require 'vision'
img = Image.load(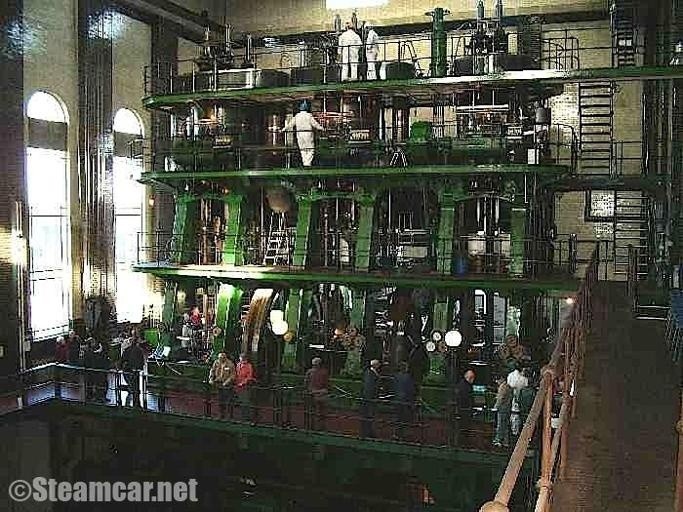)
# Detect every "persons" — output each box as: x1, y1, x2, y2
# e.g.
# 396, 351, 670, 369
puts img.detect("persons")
363, 22, 380, 79
278, 102, 326, 170
337, 24, 364, 83
56, 313, 535, 449
